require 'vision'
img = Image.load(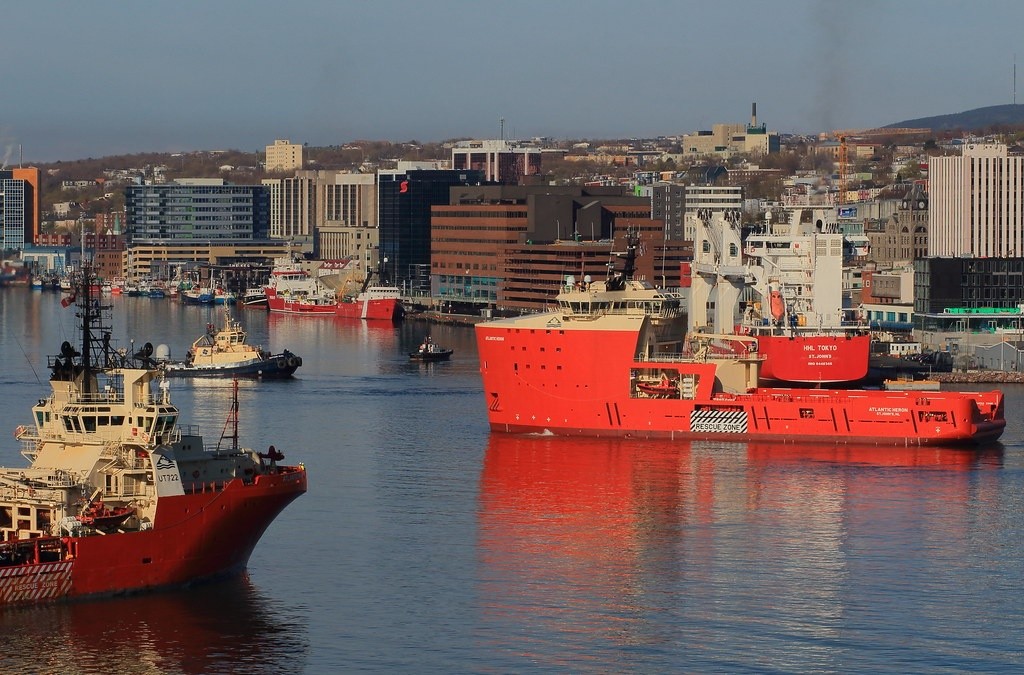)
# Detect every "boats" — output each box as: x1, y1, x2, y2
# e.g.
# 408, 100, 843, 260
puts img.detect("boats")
409, 339, 455, 360
473, 225, 1008, 446
0, 252, 403, 609
